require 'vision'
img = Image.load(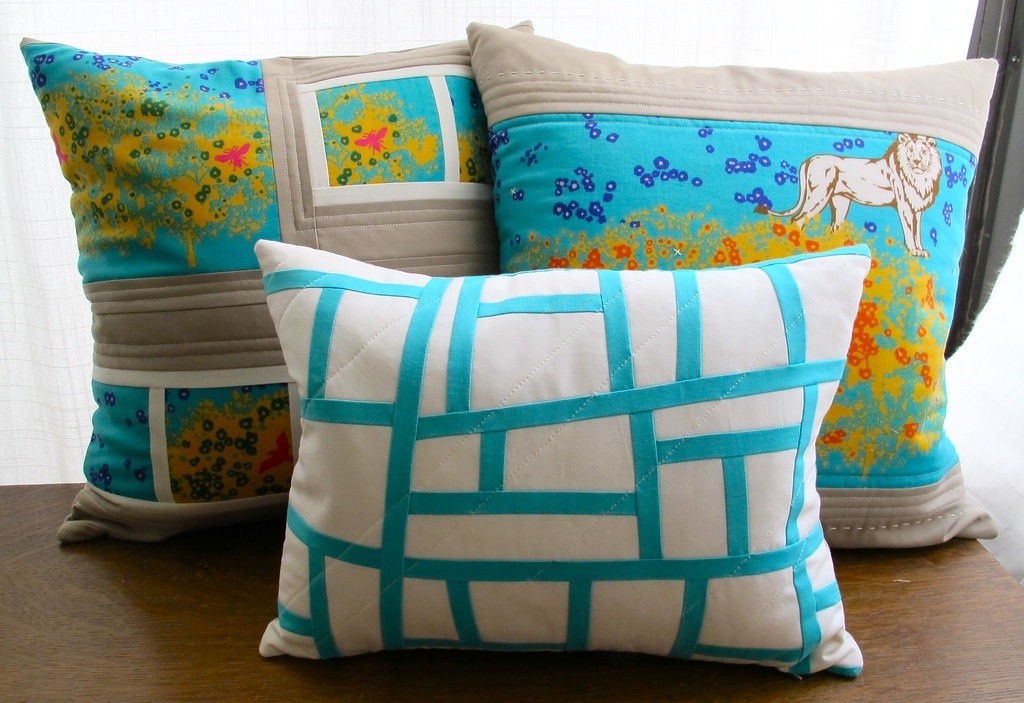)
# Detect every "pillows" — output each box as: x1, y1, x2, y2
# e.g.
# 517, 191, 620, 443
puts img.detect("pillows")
254, 238, 871, 683
16, 16, 536, 548
464, 23, 1002, 552
